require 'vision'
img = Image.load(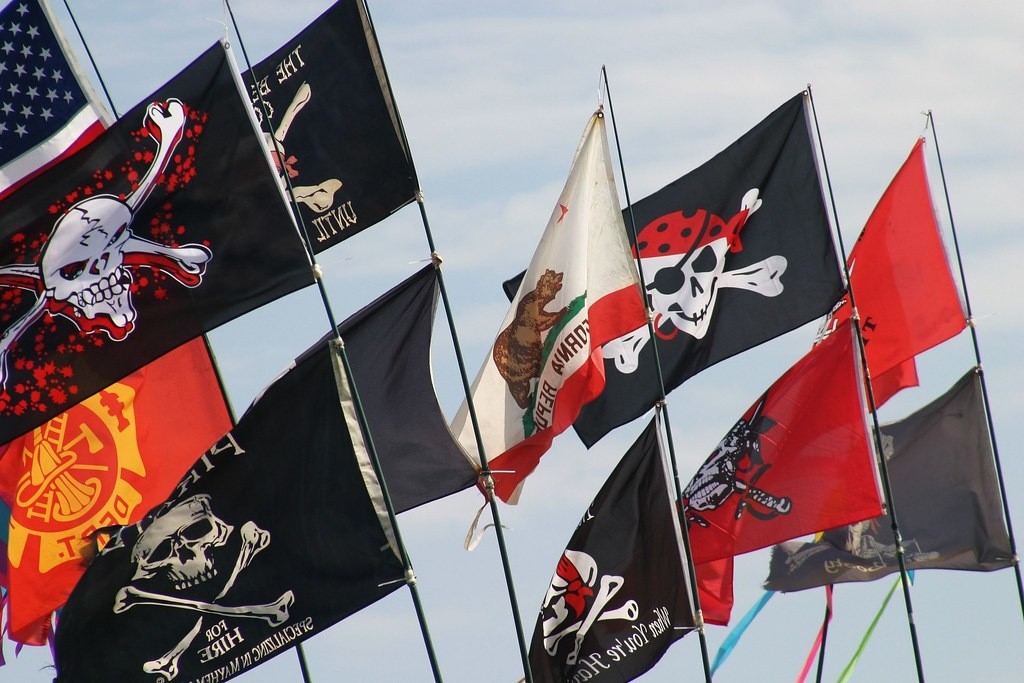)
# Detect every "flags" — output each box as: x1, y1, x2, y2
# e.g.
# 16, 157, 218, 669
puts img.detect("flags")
812, 137, 969, 413
0, 0, 115, 200
0, 334, 235, 647
240, 0, 419, 256
763, 367, 1024, 594
323, 262, 486, 515
502, 90, 846, 450
675, 319, 886, 627
450, 110, 648, 505
528, 415, 697, 683
56, 334, 406, 683
0, 39, 316, 447
